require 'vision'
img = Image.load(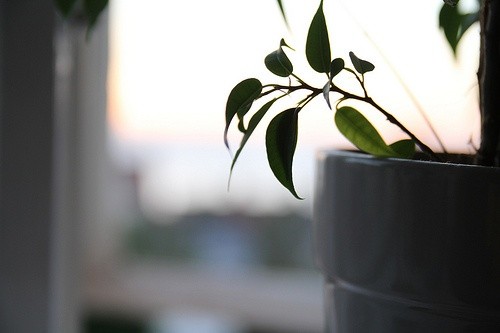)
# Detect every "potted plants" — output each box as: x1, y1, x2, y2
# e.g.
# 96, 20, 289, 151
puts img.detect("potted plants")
224, 0, 498, 333
